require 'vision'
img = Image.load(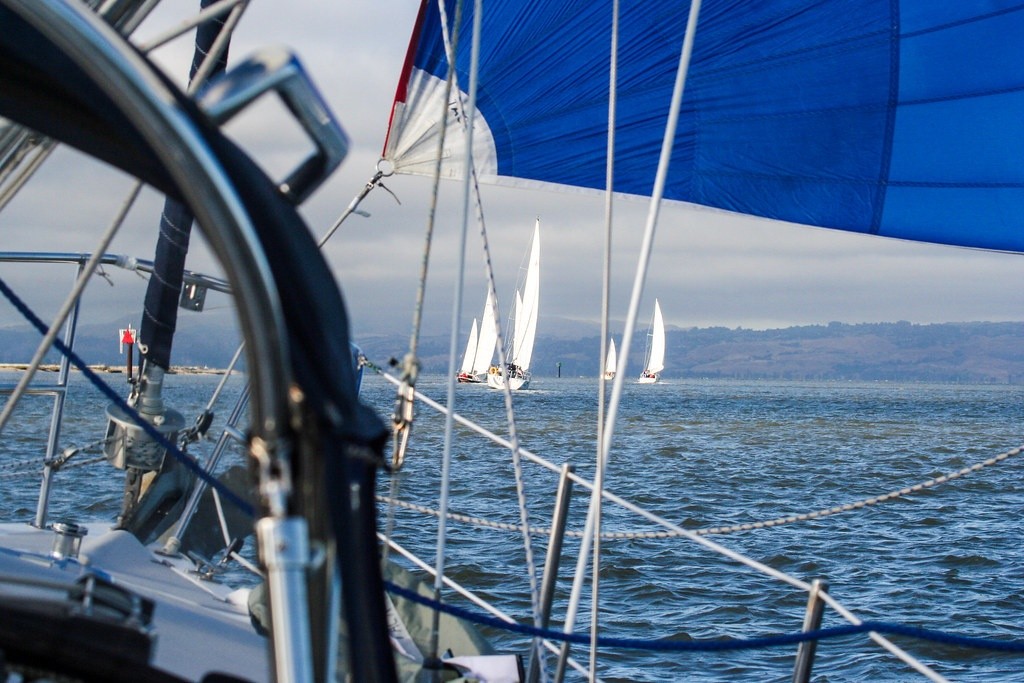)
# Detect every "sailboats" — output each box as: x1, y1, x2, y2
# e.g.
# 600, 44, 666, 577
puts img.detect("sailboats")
485, 216, 541, 390
632, 297, 666, 384
456, 288, 497, 383
604, 335, 617, 379
